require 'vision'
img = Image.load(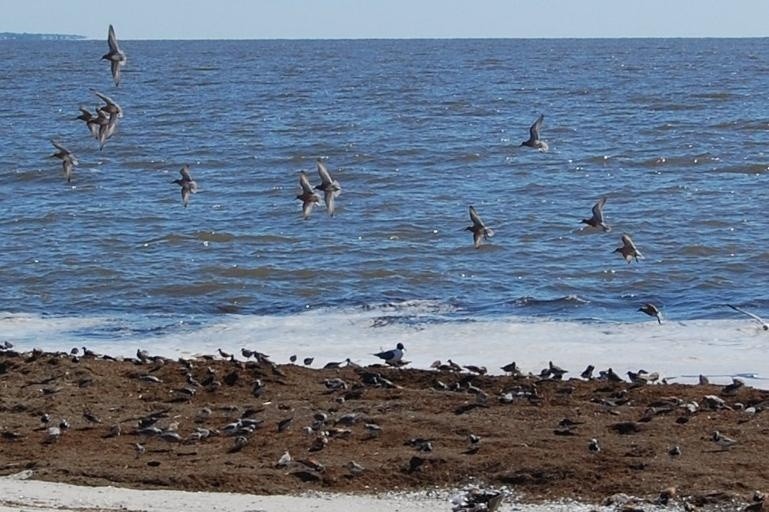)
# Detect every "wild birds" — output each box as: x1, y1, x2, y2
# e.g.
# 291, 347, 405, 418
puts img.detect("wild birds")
170, 167, 199, 208
291, 160, 342, 217
1, 342, 769, 472
40, 25, 127, 185
518, 113, 550, 154
463, 205, 495, 249
726, 303, 768, 331
636, 302, 662, 326
610, 234, 644, 265
578, 197, 612, 234
